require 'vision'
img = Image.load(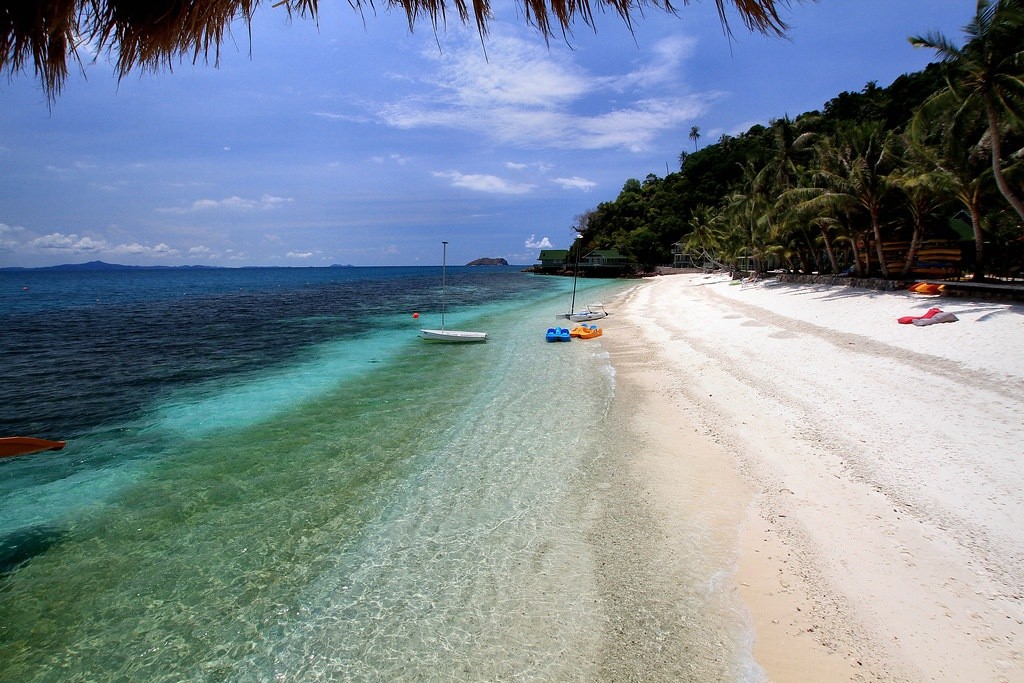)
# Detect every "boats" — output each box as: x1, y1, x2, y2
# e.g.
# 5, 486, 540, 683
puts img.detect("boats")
0, 437, 66, 459
556, 312, 606, 321
419, 328, 486, 342
